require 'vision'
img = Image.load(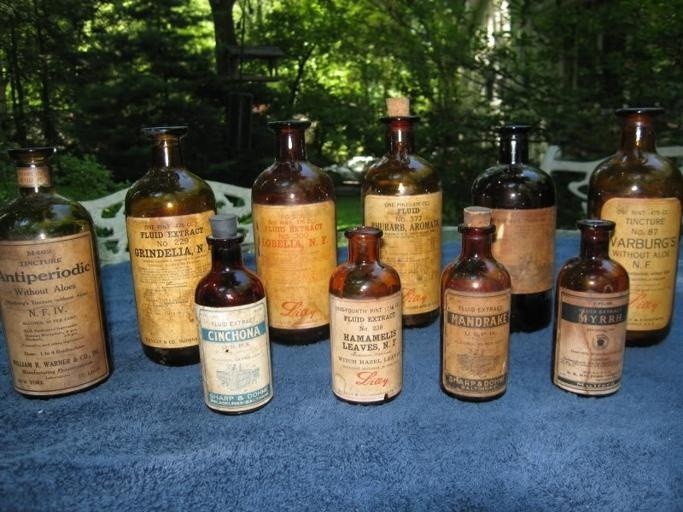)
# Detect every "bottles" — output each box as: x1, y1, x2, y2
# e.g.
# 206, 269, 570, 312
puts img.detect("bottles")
589, 108, 682, 348
251, 120, 340, 345
363, 99, 444, 329
554, 219, 630, 398
194, 217, 274, 414
442, 209, 511, 402
328, 227, 403, 405
123, 127, 217, 365
1, 145, 113, 398
472, 126, 557, 334
